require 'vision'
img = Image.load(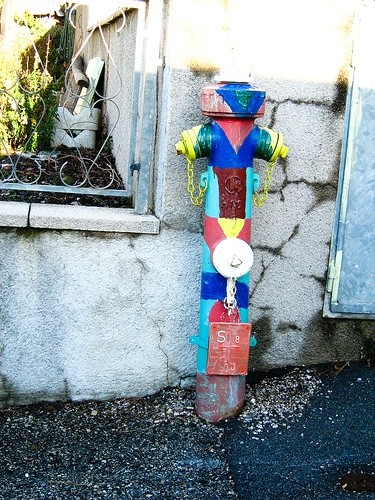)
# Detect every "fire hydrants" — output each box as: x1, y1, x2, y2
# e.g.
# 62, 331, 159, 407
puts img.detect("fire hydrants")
174, 81, 289, 424
